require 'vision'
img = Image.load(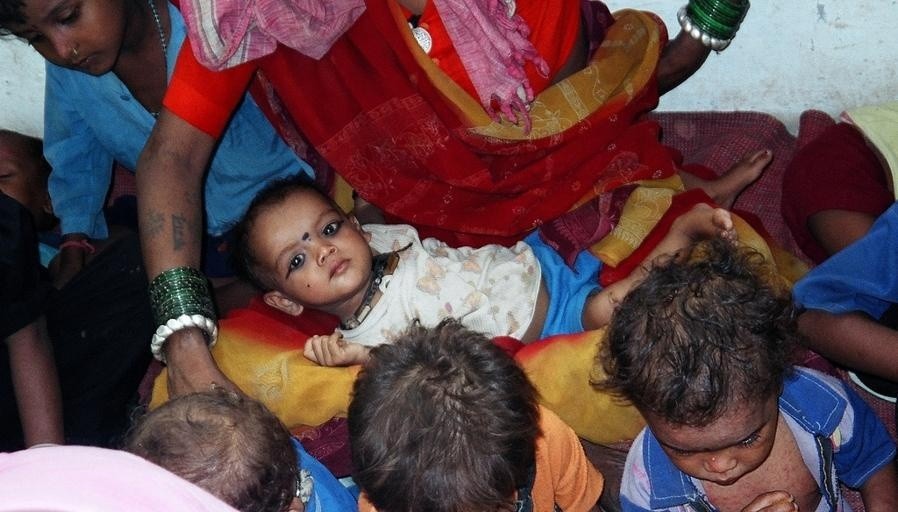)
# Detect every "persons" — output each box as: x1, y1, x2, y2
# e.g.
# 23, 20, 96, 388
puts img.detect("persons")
3, 1, 898, 512
